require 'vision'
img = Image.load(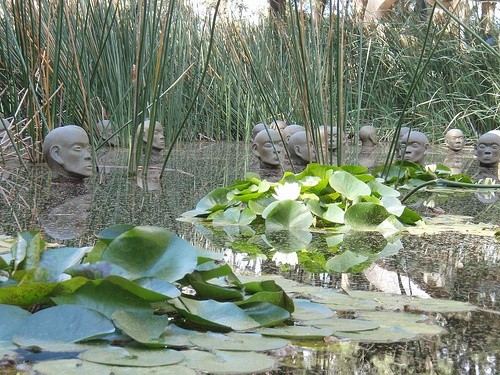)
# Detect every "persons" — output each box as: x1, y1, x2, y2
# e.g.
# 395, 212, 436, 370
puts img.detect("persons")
42, 126, 94, 185
137, 163, 163, 190
96, 147, 120, 174
39, 186, 94, 240
136, 119, 165, 163
475, 166, 500, 203
96, 120, 120, 147
253, 120, 345, 172
359, 125, 380, 148
391, 126, 412, 158
398, 131, 431, 169
357, 148, 378, 167
0, 118, 15, 156
442, 152, 466, 176
444, 129, 466, 151
474, 130, 500, 167
